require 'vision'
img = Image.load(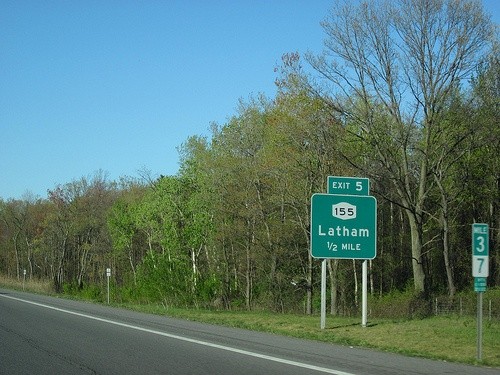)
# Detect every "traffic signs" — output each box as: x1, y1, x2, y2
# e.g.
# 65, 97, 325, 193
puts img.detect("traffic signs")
312, 175, 377, 261
